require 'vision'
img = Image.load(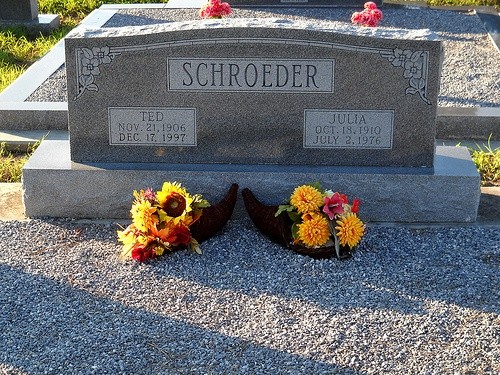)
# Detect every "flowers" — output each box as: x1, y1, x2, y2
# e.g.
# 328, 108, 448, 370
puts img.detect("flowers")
198, 0, 232, 18
278, 185, 369, 254
112, 182, 211, 264
350, 0, 384, 29
